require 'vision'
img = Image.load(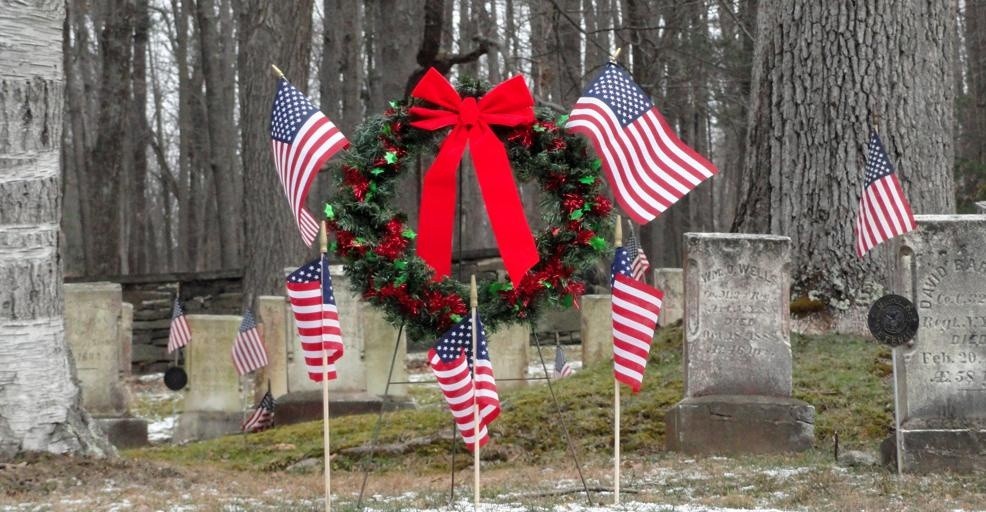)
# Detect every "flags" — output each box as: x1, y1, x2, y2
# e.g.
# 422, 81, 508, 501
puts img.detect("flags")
228, 305, 269, 376
282, 250, 346, 384
427, 306, 504, 454
266, 77, 355, 250
561, 61, 721, 228
164, 292, 193, 357
551, 346, 578, 382
620, 229, 652, 284
852, 125, 918, 260
241, 388, 280, 435
609, 245, 667, 398
